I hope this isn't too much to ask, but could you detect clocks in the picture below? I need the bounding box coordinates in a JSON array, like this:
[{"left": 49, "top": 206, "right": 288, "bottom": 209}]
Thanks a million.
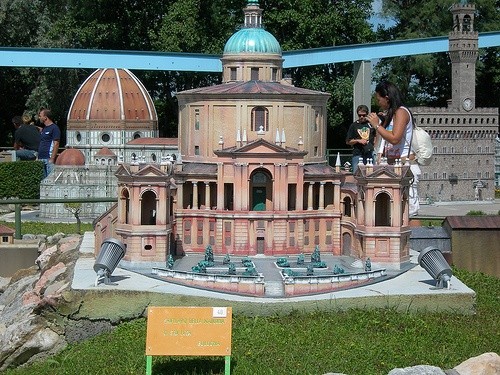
[{"left": 462, "top": 96, "right": 474, "bottom": 111}]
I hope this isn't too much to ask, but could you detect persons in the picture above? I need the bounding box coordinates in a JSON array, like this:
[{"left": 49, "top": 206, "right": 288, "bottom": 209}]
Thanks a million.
[
  {"left": 346, "top": 105, "right": 376, "bottom": 172},
  {"left": 364, "top": 80, "right": 420, "bottom": 227},
  {"left": 12, "top": 109, "right": 60, "bottom": 175}
]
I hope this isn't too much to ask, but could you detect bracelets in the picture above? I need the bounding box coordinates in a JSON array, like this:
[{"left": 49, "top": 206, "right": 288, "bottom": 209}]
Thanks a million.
[{"left": 375, "top": 124, "right": 381, "bottom": 130}]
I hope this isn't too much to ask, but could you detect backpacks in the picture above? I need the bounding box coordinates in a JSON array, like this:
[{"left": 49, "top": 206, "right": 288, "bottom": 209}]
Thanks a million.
[{"left": 393, "top": 123, "right": 433, "bottom": 166}]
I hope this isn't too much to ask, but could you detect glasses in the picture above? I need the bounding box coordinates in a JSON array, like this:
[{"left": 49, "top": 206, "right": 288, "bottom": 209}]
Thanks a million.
[{"left": 358, "top": 114, "right": 366, "bottom": 117}]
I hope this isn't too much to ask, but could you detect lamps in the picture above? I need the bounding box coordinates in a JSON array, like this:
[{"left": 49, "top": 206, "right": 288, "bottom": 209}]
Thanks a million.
[
  {"left": 418, "top": 246, "right": 454, "bottom": 291},
  {"left": 92, "top": 236, "right": 127, "bottom": 288}
]
